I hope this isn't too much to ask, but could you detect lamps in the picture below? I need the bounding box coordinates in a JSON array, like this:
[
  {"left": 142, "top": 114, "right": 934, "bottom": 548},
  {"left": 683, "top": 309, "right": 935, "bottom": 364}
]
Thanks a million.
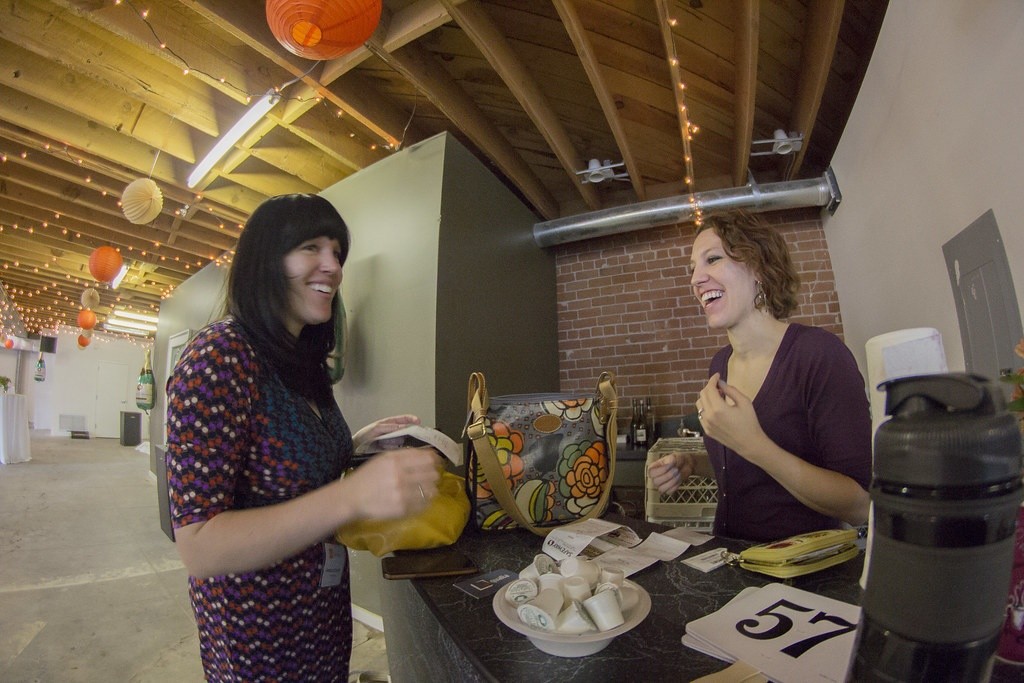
[
  {"left": 112, "top": 261, "right": 131, "bottom": 290},
  {"left": 185, "top": 86, "right": 281, "bottom": 189}
]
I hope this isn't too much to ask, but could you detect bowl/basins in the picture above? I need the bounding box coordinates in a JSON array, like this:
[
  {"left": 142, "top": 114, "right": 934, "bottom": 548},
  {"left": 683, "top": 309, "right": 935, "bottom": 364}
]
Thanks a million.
[{"left": 491, "top": 577, "right": 651, "bottom": 658}]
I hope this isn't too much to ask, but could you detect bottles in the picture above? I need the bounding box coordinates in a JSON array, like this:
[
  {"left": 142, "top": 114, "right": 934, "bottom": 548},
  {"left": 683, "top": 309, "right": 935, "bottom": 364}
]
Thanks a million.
[
  {"left": 34, "top": 351, "right": 46, "bottom": 381},
  {"left": 325, "top": 287, "right": 349, "bottom": 385},
  {"left": 645, "top": 398, "right": 656, "bottom": 446},
  {"left": 631, "top": 398, "right": 638, "bottom": 445},
  {"left": 136, "top": 349, "right": 156, "bottom": 410},
  {"left": 842, "top": 371, "right": 1023, "bottom": 683},
  {"left": 637, "top": 399, "right": 647, "bottom": 446}
]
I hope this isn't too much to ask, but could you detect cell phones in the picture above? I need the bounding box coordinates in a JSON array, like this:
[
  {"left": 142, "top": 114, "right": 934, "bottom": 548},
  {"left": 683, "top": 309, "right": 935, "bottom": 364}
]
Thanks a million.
[{"left": 381, "top": 552, "right": 477, "bottom": 580}]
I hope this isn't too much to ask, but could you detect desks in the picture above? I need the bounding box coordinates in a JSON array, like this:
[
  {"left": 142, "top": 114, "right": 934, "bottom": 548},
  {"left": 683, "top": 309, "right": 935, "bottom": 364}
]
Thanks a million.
[{"left": 0, "top": 391, "right": 32, "bottom": 465}]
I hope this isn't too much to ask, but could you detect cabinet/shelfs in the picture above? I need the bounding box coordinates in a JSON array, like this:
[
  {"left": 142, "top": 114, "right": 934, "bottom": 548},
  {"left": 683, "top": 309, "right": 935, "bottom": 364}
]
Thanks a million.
[{"left": 154, "top": 445, "right": 176, "bottom": 542}]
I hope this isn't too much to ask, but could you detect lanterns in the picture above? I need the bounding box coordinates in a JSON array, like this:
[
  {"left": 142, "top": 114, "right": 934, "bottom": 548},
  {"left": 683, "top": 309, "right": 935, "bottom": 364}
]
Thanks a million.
[
  {"left": 5, "top": 339, "right": 15, "bottom": 349},
  {"left": 78, "top": 335, "right": 91, "bottom": 347},
  {"left": 265, "top": 0, "right": 382, "bottom": 60},
  {"left": 78, "top": 310, "right": 96, "bottom": 329},
  {"left": 88, "top": 247, "right": 122, "bottom": 282}
]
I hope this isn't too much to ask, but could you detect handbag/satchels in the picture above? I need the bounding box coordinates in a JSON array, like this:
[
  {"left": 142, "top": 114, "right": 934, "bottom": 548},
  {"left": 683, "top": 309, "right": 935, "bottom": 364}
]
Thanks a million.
[
  {"left": 461, "top": 371, "right": 622, "bottom": 530},
  {"left": 335, "top": 470, "right": 469, "bottom": 556}
]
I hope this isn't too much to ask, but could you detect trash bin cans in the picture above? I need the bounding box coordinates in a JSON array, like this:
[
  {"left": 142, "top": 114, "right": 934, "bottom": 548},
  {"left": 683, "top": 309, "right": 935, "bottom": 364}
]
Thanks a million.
[{"left": 120, "top": 411, "right": 142, "bottom": 446}]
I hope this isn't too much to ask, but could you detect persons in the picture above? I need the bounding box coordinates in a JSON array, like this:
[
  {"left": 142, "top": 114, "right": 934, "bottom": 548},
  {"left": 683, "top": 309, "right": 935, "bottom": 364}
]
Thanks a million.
[
  {"left": 167, "top": 189, "right": 448, "bottom": 683},
  {"left": 648, "top": 207, "right": 873, "bottom": 542}
]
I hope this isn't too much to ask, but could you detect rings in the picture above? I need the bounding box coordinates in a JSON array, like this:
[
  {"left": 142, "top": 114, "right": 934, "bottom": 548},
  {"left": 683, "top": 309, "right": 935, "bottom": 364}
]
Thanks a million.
[{"left": 698, "top": 408, "right": 705, "bottom": 420}]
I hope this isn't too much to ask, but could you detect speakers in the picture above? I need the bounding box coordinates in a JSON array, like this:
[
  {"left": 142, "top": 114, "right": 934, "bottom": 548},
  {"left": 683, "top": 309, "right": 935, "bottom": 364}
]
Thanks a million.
[{"left": 120, "top": 411, "right": 142, "bottom": 446}]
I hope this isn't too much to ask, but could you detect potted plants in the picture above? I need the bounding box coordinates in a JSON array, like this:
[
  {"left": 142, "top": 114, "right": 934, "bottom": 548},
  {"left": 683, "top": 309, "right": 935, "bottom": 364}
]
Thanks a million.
[{"left": 0, "top": 375, "right": 12, "bottom": 394}]
